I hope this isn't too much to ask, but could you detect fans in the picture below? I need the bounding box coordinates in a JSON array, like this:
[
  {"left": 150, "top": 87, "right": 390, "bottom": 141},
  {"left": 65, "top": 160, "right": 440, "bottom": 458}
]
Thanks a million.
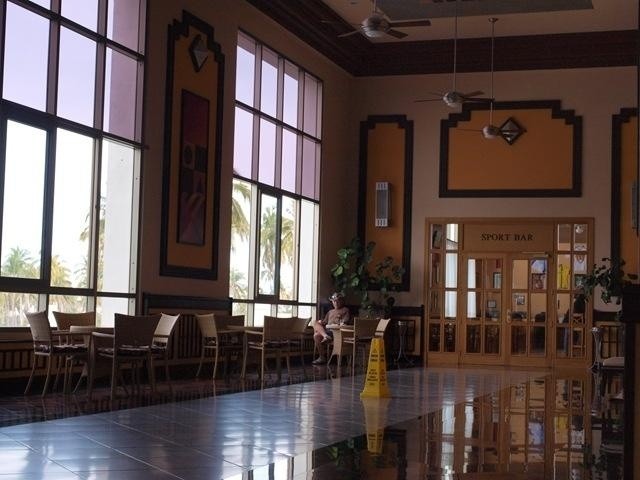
[
  {"left": 414, "top": 10, "right": 495, "bottom": 107},
  {"left": 323, "top": 0, "right": 432, "bottom": 39},
  {"left": 457, "top": 18, "right": 527, "bottom": 139}
]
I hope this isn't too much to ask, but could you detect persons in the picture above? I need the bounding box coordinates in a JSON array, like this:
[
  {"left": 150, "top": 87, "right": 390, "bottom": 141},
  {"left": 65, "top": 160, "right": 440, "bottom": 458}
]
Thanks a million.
[{"left": 310, "top": 293, "right": 350, "bottom": 365}]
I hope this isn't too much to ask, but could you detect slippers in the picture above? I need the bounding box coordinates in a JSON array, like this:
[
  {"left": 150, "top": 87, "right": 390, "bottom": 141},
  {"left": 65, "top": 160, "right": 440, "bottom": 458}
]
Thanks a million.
[{"left": 311, "top": 336, "right": 333, "bottom": 365}]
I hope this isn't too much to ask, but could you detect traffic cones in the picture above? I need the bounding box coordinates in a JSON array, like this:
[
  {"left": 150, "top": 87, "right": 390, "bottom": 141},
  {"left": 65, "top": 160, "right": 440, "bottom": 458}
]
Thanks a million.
[
  {"left": 357, "top": 400, "right": 391, "bottom": 457},
  {"left": 359, "top": 337, "right": 393, "bottom": 400}
]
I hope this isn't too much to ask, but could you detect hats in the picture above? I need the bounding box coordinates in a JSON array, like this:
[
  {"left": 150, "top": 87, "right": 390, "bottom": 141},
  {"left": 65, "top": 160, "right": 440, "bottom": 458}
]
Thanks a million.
[{"left": 328, "top": 291, "right": 345, "bottom": 302}]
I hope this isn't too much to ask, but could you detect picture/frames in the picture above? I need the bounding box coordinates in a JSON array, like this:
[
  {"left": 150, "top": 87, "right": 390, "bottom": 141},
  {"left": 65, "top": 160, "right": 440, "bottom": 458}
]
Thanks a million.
[{"left": 176, "top": 88, "right": 210, "bottom": 246}]
